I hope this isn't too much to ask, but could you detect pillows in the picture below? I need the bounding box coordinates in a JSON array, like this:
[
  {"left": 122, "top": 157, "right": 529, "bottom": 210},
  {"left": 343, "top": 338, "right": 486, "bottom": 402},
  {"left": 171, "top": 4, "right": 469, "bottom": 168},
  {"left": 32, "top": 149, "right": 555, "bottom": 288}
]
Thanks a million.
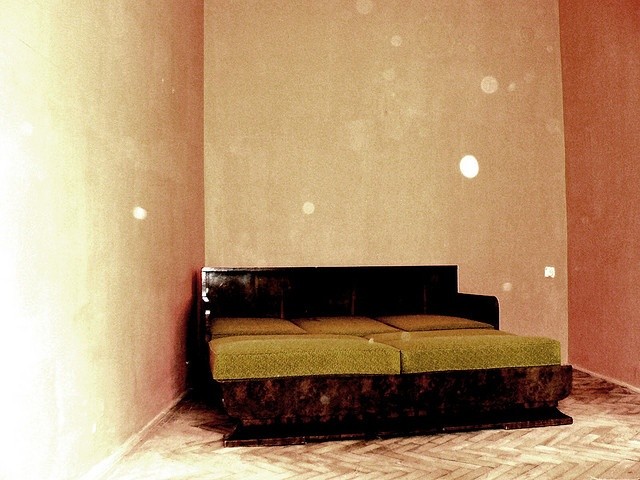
[
  {"left": 292, "top": 315, "right": 400, "bottom": 335},
  {"left": 210, "top": 315, "right": 307, "bottom": 336},
  {"left": 375, "top": 313, "right": 494, "bottom": 332}
]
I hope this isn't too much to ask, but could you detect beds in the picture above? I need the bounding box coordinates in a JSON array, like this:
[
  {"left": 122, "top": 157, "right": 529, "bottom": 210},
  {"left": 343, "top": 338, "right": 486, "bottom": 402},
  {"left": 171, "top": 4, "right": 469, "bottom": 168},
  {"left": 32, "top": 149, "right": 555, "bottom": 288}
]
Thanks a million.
[{"left": 198, "top": 265, "right": 575, "bottom": 447}]
